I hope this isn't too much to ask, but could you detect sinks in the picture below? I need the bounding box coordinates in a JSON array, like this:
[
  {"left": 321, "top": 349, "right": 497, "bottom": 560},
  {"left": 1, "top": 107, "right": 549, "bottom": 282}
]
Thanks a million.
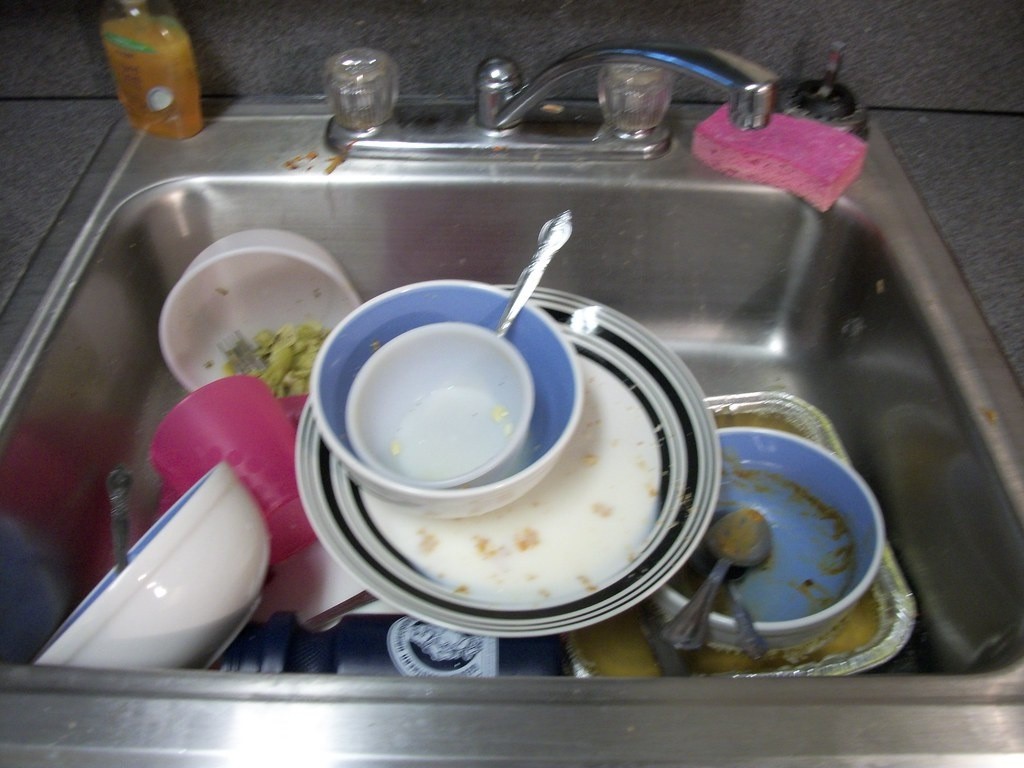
[{"left": 0, "top": 141, "right": 1024, "bottom": 719}]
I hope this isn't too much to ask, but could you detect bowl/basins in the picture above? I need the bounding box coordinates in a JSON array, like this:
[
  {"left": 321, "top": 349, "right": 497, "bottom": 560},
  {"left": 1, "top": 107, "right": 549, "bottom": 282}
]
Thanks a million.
[
  {"left": 343, "top": 321, "right": 533, "bottom": 493},
  {"left": 310, "top": 277, "right": 584, "bottom": 520},
  {"left": 157, "top": 227, "right": 360, "bottom": 399},
  {"left": 644, "top": 428, "right": 886, "bottom": 657},
  {"left": 25, "top": 459, "right": 274, "bottom": 674}
]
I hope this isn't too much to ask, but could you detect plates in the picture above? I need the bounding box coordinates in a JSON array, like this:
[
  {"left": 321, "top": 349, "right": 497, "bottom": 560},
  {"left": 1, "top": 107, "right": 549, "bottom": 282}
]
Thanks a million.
[{"left": 295, "top": 283, "right": 721, "bottom": 641}]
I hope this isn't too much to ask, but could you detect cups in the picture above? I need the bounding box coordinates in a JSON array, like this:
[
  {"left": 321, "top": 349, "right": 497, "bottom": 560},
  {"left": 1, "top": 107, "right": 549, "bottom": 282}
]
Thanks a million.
[{"left": 148, "top": 375, "right": 322, "bottom": 554}]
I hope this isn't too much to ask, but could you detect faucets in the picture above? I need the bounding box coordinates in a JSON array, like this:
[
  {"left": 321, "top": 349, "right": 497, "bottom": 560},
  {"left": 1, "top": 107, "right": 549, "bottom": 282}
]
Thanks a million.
[{"left": 472, "top": 38, "right": 782, "bottom": 143}]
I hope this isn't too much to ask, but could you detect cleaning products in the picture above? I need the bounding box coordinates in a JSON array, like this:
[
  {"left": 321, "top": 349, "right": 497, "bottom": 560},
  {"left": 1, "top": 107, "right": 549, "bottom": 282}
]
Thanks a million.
[{"left": 99, "top": 0, "right": 206, "bottom": 140}]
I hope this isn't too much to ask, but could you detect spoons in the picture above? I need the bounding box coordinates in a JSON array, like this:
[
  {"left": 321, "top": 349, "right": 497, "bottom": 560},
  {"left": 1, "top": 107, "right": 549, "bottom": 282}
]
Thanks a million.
[{"left": 655, "top": 507, "right": 770, "bottom": 653}]
